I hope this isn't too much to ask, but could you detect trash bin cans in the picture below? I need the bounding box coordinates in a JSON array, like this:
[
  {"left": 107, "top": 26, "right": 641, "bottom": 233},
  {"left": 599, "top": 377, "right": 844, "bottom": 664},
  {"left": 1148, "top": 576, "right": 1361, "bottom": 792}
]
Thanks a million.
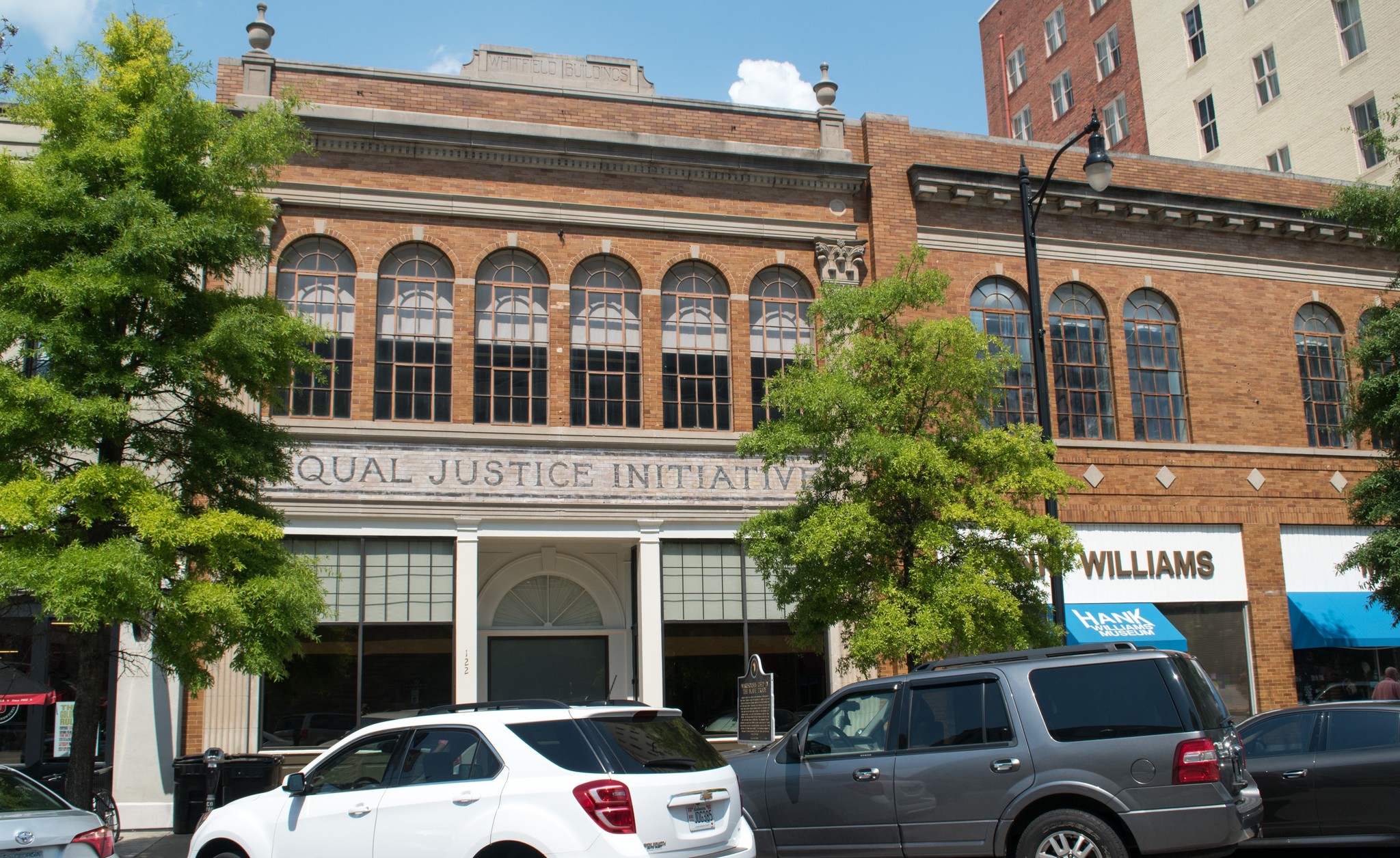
[
  {"left": 172, "top": 753, "right": 224, "bottom": 834},
  {"left": 220, "top": 752, "right": 285, "bottom": 806}
]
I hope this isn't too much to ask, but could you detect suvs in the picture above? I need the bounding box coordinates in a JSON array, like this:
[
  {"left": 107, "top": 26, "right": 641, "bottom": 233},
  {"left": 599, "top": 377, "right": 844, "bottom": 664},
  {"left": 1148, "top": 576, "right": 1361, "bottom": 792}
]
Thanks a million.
[
  {"left": 720, "top": 639, "right": 1265, "bottom": 858},
  {"left": 183, "top": 699, "right": 761, "bottom": 858}
]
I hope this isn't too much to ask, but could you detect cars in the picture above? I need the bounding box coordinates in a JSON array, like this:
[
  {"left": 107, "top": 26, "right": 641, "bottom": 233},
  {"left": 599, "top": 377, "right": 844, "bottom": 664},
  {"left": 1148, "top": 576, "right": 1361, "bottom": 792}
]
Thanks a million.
[
  {"left": 1305, "top": 680, "right": 1382, "bottom": 702},
  {"left": 1, "top": 763, "right": 121, "bottom": 858},
  {"left": 1217, "top": 699, "right": 1400, "bottom": 858}
]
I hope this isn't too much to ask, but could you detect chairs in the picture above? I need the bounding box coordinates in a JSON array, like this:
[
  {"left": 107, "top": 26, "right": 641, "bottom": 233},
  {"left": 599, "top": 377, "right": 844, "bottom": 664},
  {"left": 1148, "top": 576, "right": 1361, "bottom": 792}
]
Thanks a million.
[{"left": 911, "top": 698, "right": 935, "bottom": 748}]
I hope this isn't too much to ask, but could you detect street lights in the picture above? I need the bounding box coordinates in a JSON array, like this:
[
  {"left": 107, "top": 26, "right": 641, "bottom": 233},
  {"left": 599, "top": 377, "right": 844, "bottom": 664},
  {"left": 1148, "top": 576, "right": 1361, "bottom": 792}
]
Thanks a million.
[{"left": 1017, "top": 107, "right": 1117, "bottom": 645}]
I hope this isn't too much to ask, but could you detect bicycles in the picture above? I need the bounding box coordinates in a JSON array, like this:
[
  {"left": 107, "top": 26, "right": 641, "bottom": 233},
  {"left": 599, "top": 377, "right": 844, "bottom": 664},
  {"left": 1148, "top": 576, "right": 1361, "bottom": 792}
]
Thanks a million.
[{"left": 42, "top": 769, "right": 121, "bottom": 844}]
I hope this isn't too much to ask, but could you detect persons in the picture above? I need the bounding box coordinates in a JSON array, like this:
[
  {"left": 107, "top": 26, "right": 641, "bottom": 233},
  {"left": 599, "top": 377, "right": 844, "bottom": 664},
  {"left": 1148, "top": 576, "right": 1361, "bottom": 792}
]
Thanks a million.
[{"left": 1371, "top": 667, "right": 1400, "bottom": 700}]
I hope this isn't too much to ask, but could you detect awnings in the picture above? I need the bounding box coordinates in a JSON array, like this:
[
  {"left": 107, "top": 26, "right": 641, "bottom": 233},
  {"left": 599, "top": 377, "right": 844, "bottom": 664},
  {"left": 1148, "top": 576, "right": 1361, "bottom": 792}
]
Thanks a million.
[
  {"left": 1287, "top": 592, "right": 1400, "bottom": 649},
  {"left": 1047, "top": 603, "right": 1188, "bottom": 652}
]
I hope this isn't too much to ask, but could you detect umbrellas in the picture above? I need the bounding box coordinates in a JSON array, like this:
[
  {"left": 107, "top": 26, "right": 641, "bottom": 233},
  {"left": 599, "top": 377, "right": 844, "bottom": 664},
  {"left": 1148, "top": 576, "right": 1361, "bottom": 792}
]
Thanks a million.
[{"left": 0, "top": 661, "right": 56, "bottom": 705}]
